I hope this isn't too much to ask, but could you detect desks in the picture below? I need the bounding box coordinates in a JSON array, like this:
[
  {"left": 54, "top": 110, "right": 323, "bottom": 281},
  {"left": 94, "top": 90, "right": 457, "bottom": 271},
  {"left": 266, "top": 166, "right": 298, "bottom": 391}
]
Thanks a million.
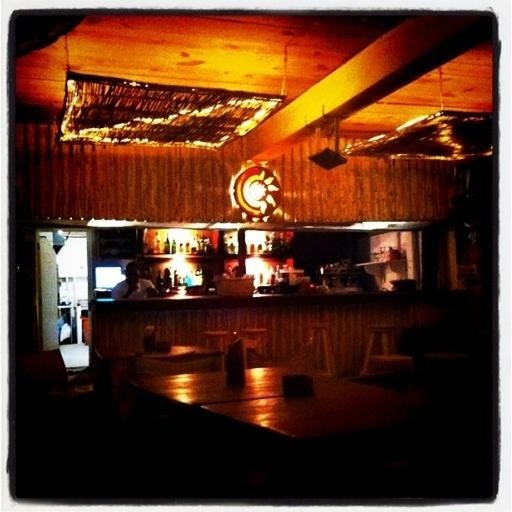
[
  {"left": 127, "top": 366, "right": 301, "bottom": 407},
  {"left": 201, "top": 382, "right": 416, "bottom": 440},
  {"left": 139, "top": 345, "right": 196, "bottom": 360}
]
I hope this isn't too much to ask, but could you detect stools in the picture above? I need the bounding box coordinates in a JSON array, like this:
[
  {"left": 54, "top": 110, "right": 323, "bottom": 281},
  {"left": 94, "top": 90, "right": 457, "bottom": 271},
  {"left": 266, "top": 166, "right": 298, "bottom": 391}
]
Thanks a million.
[
  {"left": 203, "top": 322, "right": 337, "bottom": 381},
  {"left": 363, "top": 324, "right": 400, "bottom": 372}
]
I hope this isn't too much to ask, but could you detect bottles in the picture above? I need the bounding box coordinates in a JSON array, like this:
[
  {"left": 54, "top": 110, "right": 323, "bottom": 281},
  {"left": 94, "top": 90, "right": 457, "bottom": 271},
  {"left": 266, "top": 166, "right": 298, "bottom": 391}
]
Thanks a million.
[
  {"left": 156, "top": 267, "right": 179, "bottom": 291},
  {"left": 153, "top": 231, "right": 190, "bottom": 255},
  {"left": 276, "top": 264, "right": 296, "bottom": 285}
]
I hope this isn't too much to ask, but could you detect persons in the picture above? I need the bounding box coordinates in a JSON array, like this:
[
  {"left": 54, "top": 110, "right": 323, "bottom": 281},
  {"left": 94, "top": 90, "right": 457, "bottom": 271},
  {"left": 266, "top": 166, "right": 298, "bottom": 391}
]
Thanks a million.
[
  {"left": 111, "top": 261, "right": 160, "bottom": 300},
  {"left": 232, "top": 266, "right": 243, "bottom": 278}
]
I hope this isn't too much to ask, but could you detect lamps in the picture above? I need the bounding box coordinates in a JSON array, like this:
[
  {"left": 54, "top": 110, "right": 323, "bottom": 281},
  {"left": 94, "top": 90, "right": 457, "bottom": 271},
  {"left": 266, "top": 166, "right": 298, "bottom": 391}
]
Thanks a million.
[{"left": 232, "top": 167, "right": 282, "bottom": 219}]
